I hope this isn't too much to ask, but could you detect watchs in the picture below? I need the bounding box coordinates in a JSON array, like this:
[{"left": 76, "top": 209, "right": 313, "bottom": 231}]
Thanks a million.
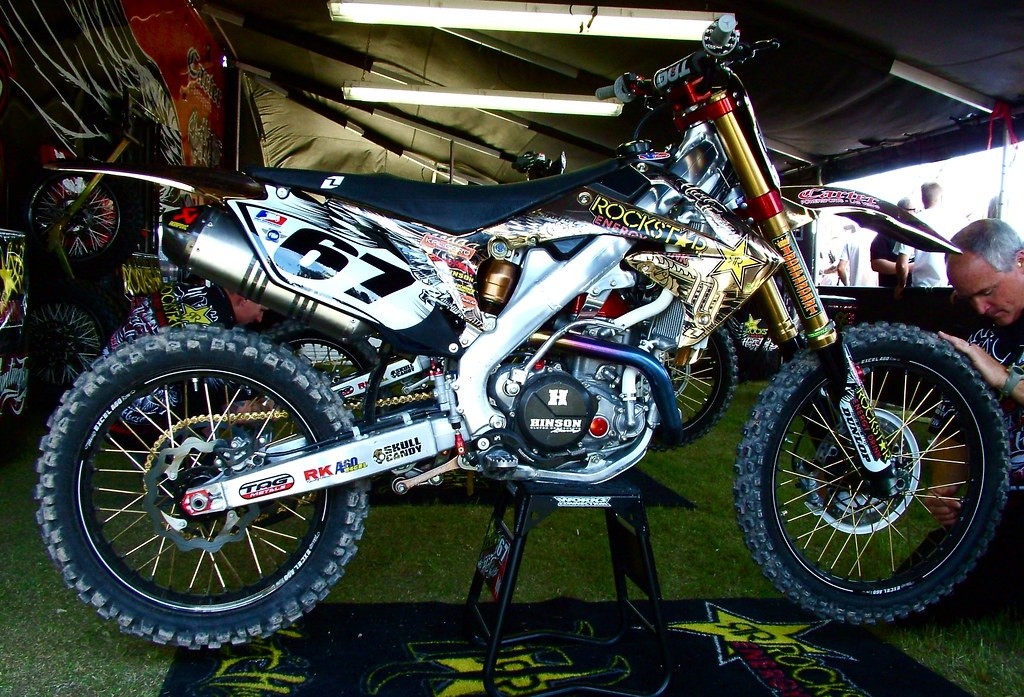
[{"left": 999, "top": 364, "right": 1024, "bottom": 398}]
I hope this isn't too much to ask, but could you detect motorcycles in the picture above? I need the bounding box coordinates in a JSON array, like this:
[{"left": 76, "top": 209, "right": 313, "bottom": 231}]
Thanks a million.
[{"left": 29, "top": 8, "right": 1013, "bottom": 697}]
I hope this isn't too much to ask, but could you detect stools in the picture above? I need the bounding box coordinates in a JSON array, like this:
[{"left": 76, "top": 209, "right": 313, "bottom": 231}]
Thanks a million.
[{"left": 460, "top": 465, "right": 698, "bottom": 697}]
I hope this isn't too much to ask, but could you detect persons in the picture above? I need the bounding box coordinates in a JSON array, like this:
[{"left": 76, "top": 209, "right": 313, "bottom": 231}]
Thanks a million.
[
  {"left": 889, "top": 219, "right": 1024, "bottom": 625},
  {"left": 815, "top": 183, "right": 962, "bottom": 305}
]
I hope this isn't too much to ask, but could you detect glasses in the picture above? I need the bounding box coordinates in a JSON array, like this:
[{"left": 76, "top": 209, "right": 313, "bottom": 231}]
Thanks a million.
[{"left": 906, "top": 208, "right": 922, "bottom": 213}]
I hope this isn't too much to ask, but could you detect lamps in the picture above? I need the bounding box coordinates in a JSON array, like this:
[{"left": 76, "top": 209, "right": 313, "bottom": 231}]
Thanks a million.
[
  {"left": 343, "top": 23, "right": 624, "bottom": 117},
  {"left": 328, "top": 0, "right": 737, "bottom": 41}
]
